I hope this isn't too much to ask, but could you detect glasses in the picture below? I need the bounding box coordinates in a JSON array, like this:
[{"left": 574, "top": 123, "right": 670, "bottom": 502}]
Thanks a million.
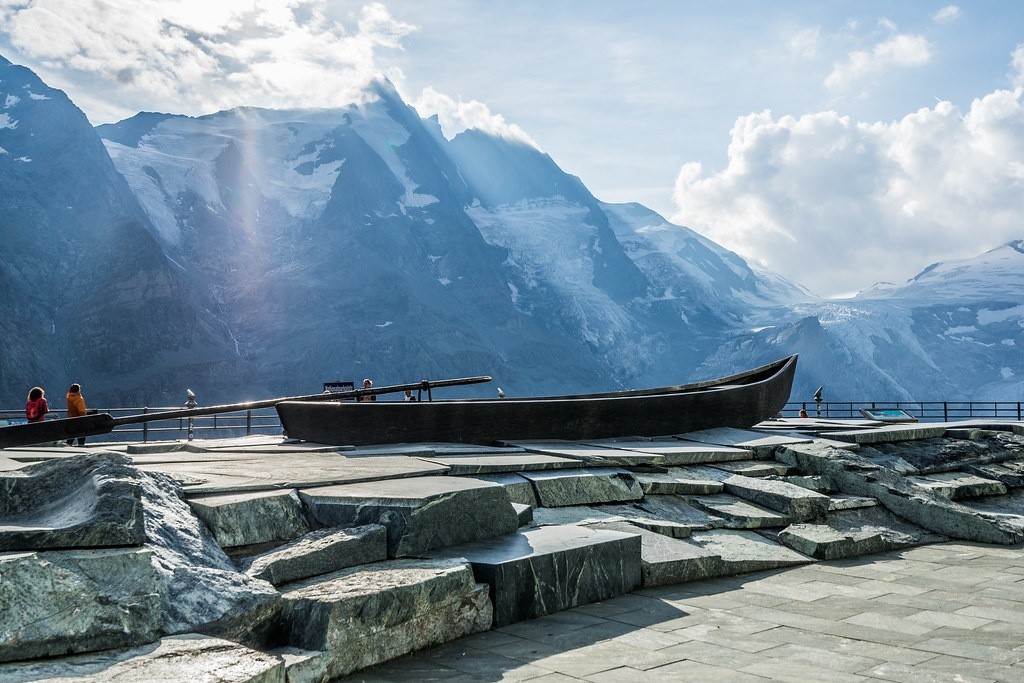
[{"left": 364, "top": 382, "right": 370, "bottom": 384}]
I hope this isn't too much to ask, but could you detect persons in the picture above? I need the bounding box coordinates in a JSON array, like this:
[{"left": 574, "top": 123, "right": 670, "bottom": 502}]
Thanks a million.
[
  {"left": 26, "top": 387, "right": 49, "bottom": 423},
  {"left": 66, "top": 384, "right": 87, "bottom": 446},
  {"left": 404, "top": 390, "right": 416, "bottom": 401},
  {"left": 799, "top": 410, "right": 808, "bottom": 418},
  {"left": 360, "top": 379, "right": 376, "bottom": 401}
]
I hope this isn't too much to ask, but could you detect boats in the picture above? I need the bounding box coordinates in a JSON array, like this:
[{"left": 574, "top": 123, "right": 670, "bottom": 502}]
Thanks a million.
[{"left": 273, "top": 351, "right": 802, "bottom": 446}]
